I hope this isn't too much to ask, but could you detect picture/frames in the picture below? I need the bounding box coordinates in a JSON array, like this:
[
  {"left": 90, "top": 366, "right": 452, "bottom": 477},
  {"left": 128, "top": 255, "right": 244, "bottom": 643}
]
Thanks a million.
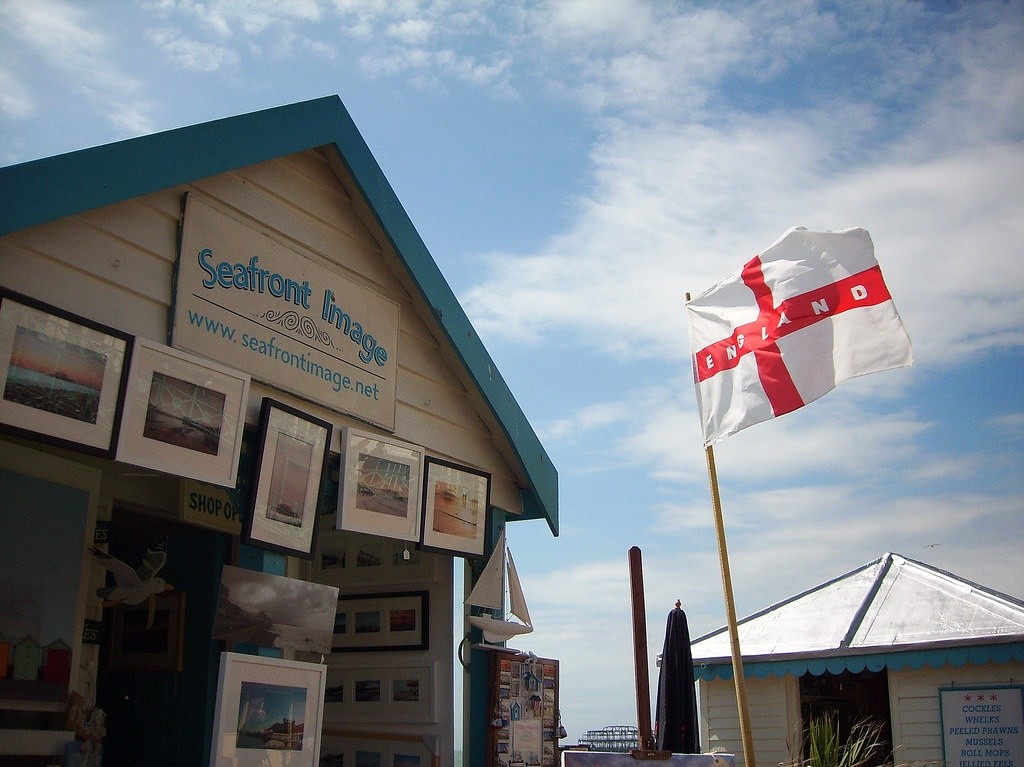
[
  {"left": 0, "top": 286, "right": 137, "bottom": 461},
  {"left": 336, "top": 426, "right": 425, "bottom": 543},
  {"left": 304, "top": 530, "right": 440, "bottom": 588},
  {"left": 240, "top": 397, "right": 334, "bottom": 562},
  {"left": 322, "top": 660, "right": 438, "bottom": 724},
  {"left": 105, "top": 589, "right": 187, "bottom": 675},
  {"left": 330, "top": 590, "right": 430, "bottom": 652},
  {"left": 209, "top": 651, "right": 328, "bottom": 767},
  {"left": 485, "top": 651, "right": 559, "bottom": 767},
  {"left": 318, "top": 728, "right": 441, "bottom": 767},
  {"left": 416, "top": 455, "right": 491, "bottom": 563},
  {"left": 112, "top": 335, "right": 250, "bottom": 489}
]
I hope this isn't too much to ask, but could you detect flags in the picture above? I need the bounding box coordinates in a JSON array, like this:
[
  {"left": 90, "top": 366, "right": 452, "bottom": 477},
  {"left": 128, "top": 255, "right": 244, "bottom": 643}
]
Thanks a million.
[{"left": 686, "top": 222, "right": 912, "bottom": 450}]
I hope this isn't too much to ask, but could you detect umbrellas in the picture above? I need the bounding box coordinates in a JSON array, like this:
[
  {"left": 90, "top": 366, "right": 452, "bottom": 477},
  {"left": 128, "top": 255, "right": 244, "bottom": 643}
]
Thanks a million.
[{"left": 655, "top": 600, "right": 700, "bottom": 755}]
